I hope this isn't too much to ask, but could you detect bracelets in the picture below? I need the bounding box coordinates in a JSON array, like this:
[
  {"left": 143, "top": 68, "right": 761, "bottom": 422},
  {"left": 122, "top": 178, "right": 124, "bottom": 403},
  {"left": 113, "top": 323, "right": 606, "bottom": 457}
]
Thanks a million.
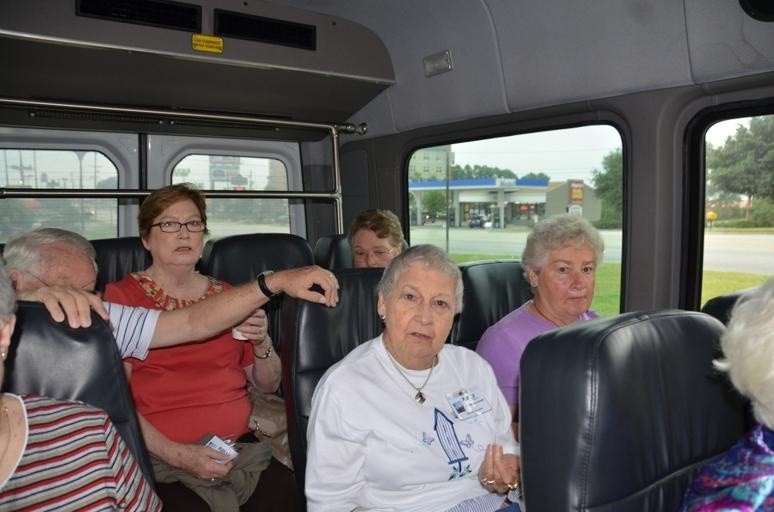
[{"left": 253, "top": 345, "right": 274, "bottom": 359}]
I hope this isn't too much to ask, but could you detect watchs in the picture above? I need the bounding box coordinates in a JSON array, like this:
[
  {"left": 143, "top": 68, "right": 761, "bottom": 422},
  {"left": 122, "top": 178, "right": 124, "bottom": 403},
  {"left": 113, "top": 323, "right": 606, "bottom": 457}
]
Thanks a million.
[{"left": 256, "top": 270, "right": 281, "bottom": 301}]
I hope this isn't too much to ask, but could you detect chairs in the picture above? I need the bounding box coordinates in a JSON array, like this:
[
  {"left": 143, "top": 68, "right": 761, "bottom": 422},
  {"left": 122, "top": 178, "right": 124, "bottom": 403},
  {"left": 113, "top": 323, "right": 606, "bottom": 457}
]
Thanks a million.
[
  {"left": 4, "top": 300, "right": 158, "bottom": 492},
  {"left": 703, "top": 289, "right": 747, "bottom": 323},
  {"left": 90, "top": 237, "right": 151, "bottom": 292},
  {"left": 454, "top": 259, "right": 534, "bottom": 351},
  {"left": 520, "top": 307, "right": 755, "bottom": 512},
  {"left": 202, "top": 234, "right": 315, "bottom": 352},
  {"left": 314, "top": 236, "right": 410, "bottom": 267},
  {"left": 280, "top": 268, "right": 454, "bottom": 481}
]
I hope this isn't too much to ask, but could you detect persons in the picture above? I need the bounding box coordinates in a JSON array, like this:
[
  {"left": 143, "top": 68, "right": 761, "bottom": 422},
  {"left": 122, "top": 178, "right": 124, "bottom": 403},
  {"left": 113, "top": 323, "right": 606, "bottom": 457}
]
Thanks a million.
[
  {"left": 680, "top": 275, "right": 774, "bottom": 512},
  {"left": 303, "top": 243, "right": 527, "bottom": 512},
  {"left": 473, "top": 211, "right": 606, "bottom": 442},
  {"left": 346, "top": 205, "right": 404, "bottom": 269},
  {"left": 0, "top": 255, "right": 164, "bottom": 512},
  {"left": 100, "top": 182, "right": 304, "bottom": 512},
  {"left": 2, "top": 227, "right": 340, "bottom": 363}
]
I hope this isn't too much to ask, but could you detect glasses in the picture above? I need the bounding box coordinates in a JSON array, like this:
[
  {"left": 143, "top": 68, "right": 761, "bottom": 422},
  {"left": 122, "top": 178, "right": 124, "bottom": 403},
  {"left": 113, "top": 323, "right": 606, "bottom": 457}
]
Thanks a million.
[{"left": 151, "top": 219, "right": 206, "bottom": 233}]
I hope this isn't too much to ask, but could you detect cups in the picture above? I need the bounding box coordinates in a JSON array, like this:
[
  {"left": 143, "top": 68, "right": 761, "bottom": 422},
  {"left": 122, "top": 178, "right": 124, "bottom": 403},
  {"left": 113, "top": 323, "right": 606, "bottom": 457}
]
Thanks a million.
[{"left": 231, "top": 320, "right": 250, "bottom": 342}]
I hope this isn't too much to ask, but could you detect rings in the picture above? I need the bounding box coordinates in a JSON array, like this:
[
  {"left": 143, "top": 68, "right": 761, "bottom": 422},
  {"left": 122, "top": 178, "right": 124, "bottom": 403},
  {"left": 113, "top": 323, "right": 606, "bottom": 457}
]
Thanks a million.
[
  {"left": 211, "top": 478, "right": 214, "bottom": 482},
  {"left": 507, "top": 483, "right": 519, "bottom": 491},
  {"left": 487, "top": 480, "right": 496, "bottom": 488},
  {"left": 480, "top": 478, "right": 488, "bottom": 487},
  {"left": 328, "top": 273, "right": 334, "bottom": 278}
]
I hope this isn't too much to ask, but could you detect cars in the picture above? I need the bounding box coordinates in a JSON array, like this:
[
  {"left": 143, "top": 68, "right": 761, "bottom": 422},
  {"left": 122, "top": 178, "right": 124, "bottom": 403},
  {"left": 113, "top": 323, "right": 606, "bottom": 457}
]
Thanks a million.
[{"left": 469, "top": 216, "right": 485, "bottom": 228}]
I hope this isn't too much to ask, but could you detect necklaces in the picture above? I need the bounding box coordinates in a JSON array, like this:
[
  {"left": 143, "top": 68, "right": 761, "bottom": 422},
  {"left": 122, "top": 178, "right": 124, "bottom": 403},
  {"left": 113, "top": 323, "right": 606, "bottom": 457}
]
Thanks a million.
[{"left": 381, "top": 333, "right": 437, "bottom": 404}]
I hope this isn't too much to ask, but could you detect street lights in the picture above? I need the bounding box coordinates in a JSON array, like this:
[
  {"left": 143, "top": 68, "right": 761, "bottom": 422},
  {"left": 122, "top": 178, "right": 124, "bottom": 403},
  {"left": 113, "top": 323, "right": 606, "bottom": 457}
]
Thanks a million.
[{"left": 75, "top": 151, "right": 88, "bottom": 236}]
